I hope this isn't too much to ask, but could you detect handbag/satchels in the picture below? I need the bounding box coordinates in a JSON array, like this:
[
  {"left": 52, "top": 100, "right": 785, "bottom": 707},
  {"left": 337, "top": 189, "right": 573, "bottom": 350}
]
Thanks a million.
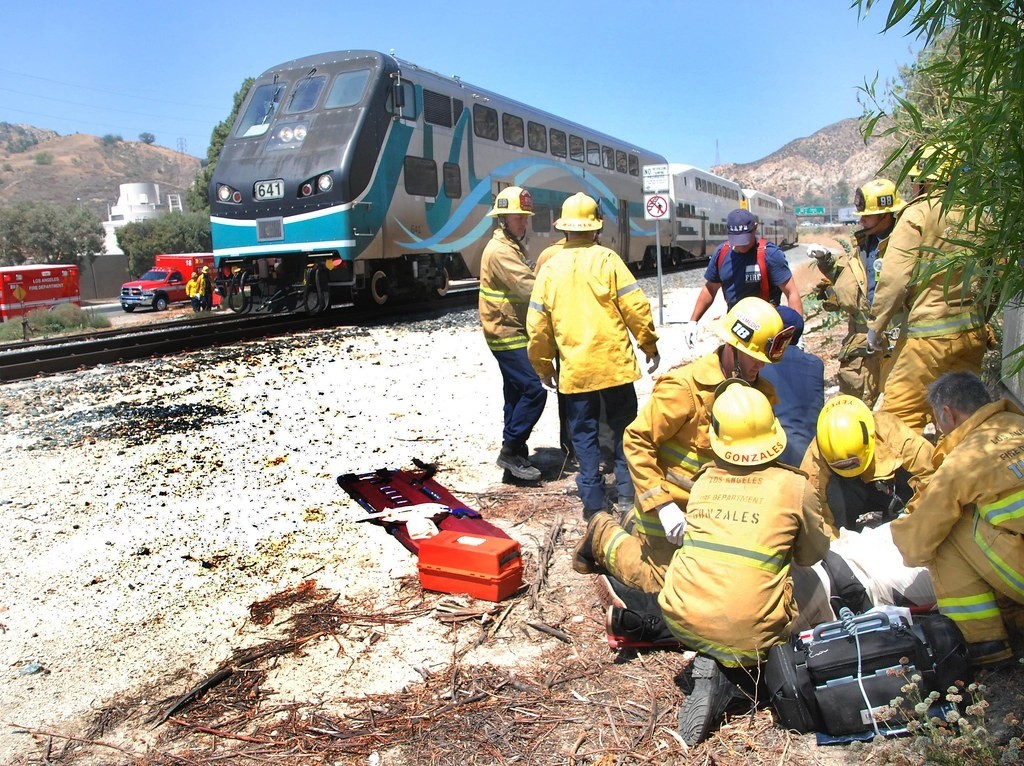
[{"left": 762, "top": 612, "right": 977, "bottom": 745}]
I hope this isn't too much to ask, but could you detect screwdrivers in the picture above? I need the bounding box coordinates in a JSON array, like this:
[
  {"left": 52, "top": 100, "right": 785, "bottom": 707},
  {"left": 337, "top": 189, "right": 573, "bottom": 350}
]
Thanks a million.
[
  {"left": 350, "top": 491, "right": 374, "bottom": 513},
  {"left": 395, "top": 468, "right": 441, "bottom": 500}
]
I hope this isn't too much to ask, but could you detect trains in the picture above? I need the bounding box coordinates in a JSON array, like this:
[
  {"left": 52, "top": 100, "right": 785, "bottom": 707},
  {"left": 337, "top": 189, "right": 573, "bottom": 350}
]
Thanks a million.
[
  {"left": 837, "top": 207, "right": 859, "bottom": 225},
  {"left": 209, "top": 49, "right": 799, "bottom": 320}
]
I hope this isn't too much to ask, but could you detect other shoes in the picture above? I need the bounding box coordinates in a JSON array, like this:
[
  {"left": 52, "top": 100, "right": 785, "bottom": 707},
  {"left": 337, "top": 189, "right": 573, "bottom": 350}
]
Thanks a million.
[
  {"left": 968, "top": 641, "right": 1014, "bottom": 666},
  {"left": 497, "top": 434, "right": 685, "bottom": 647}
]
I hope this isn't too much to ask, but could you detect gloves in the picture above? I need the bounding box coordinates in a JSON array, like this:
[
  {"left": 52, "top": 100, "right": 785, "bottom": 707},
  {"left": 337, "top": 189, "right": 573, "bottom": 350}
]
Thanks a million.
[
  {"left": 684, "top": 321, "right": 697, "bottom": 350},
  {"left": 655, "top": 500, "right": 687, "bottom": 547},
  {"left": 806, "top": 243, "right": 828, "bottom": 258},
  {"left": 866, "top": 329, "right": 885, "bottom": 350},
  {"left": 540, "top": 367, "right": 560, "bottom": 394},
  {"left": 646, "top": 352, "right": 660, "bottom": 374}
]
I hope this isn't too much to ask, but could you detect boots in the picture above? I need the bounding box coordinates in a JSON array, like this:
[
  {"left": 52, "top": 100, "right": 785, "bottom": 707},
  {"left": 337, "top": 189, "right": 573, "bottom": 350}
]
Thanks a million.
[{"left": 679, "top": 651, "right": 769, "bottom": 747}]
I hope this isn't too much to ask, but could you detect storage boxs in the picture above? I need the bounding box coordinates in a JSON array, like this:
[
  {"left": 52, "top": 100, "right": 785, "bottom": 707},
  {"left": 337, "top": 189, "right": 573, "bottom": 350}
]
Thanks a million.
[{"left": 418, "top": 528, "right": 525, "bottom": 603}]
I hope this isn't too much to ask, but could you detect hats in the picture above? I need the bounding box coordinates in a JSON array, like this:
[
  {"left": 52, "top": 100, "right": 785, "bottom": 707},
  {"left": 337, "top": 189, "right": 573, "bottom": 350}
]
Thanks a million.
[
  {"left": 725, "top": 209, "right": 755, "bottom": 247},
  {"left": 775, "top": 305, "right": 805, "bottom": 345}
]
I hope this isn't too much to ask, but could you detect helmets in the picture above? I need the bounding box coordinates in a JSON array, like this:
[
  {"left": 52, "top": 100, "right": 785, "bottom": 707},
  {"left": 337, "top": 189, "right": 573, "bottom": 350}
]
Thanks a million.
[
  {"left": 708, "top": 377, "right": 787, "bottom": 467},
  {"left": 707, "top": 297, "right": 795, "bottom": 364},
  {"left": 554, "top": 192, "right": 603, "bottom": 232},
  {"left": 485, "top": 185, "right": 536, "bottom": 217},
  {"left": 201, "top": 266, "right": 210, "bottom": 274},
  {"left": 852, "top": 179, "right": 909, "bottom": 216},
  {"left": 904, "top": 140, "right": 963, "bottom": 182},
  {"left": 191, "top": 272, "right": 198, "bottom": 279},
  {"left": 816, "top": 395, "right": 875, "bottom": 478}
]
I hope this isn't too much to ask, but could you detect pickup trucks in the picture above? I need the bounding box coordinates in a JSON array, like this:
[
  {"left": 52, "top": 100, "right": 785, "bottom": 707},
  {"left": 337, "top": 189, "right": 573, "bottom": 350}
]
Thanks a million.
[{"left": 120, "top": 253, "right": 230, "bottom": 313}]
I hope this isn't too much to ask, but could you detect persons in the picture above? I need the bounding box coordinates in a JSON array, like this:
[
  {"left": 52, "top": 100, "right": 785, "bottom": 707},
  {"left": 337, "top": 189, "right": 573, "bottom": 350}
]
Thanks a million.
[
  {"left": 686, "top": 209, "right": 804, "bottom": 350},
  {"left": 889, "top": 372, "right": 1023, "bottom": 668},
  {"left": 806, "top": 178, "right": 907, "bottom": 398},
  {"left": 527, "top": 193, "right": 662, "bottom": 523},
  {"left": 867, "top": 143, "right": 1005, "bottom": 438},
  {"left": 572, "top": 297, "right": 829, "bottom": 738},
  {"left": 478, "top": 188, "right": 542, "bottom": 480},
  {"left": 186, "top": 266, "right": 214, "bottom": 313},
  {"left": 800, "top": 394, "right": 935, "bottom": 539},
  {"left": 760, "top": 305, "right": 823, "bottom": 468}
]
